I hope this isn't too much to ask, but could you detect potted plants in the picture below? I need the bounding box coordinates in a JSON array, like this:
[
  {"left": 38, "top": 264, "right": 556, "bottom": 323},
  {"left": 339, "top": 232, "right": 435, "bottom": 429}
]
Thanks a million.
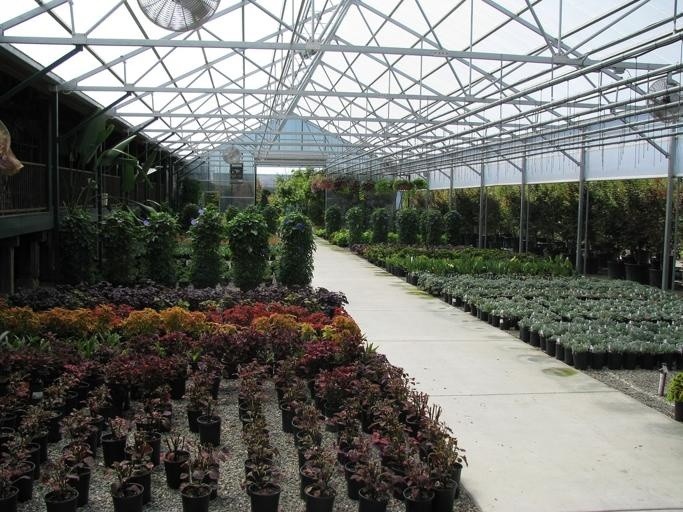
[
  {"left": 172, "top": 242, "right": 277, "bottom": 262},
  {"left": 473, "top": 180, "right": 683, "bottom": 291},
  {"left": 666, "top": 373, "right": 683, "bottom": 423}
]
